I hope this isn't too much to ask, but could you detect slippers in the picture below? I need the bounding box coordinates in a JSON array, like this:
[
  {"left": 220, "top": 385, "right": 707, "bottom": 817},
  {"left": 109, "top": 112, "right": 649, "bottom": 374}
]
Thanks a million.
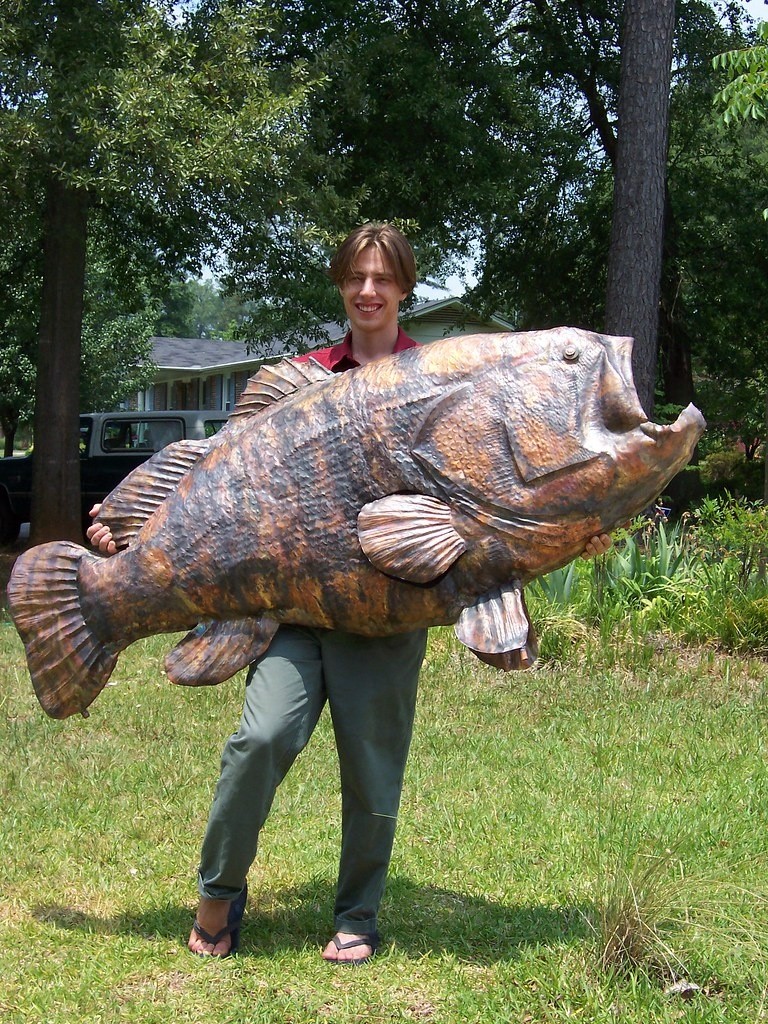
[
  {"left": 193, "top": 876, "right": 248, "bottom": 959},
  {"left": 323, "top": 934, "right": 378, "bottom": 967}
]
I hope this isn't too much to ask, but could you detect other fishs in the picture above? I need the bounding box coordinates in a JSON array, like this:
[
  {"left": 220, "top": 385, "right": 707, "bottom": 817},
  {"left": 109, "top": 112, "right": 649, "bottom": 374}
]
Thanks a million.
[{"left": 6, "top": 326, "right": 707, "bottom": 719}]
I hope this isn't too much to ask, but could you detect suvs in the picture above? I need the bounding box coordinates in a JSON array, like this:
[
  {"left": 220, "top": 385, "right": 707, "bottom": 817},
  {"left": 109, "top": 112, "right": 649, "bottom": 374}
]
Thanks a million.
[{"left": 0, "top": 407, "right": 234, "bottom": 550}]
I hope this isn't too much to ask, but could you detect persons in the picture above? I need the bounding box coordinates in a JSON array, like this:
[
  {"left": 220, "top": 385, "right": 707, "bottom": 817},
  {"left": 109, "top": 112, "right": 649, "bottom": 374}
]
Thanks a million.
[{"left": 86, "top": 225, "right": 613, "bottom": 961}]
